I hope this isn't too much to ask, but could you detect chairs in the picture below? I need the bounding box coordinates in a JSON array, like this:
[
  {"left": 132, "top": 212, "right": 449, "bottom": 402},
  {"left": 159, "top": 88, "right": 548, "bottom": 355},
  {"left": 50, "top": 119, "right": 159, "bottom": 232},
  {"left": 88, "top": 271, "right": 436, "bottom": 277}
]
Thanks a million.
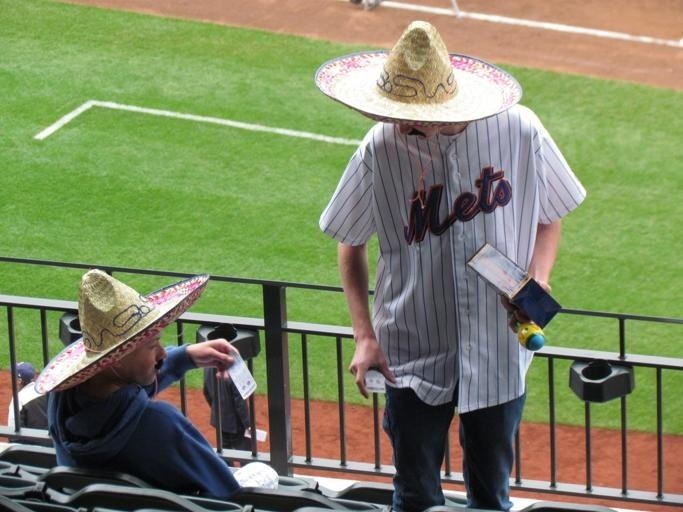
[
  {"left": 224, "top": 477, "right": 467, "bottom": 512},
  {"left": 526, "top": 501, "right": 612, "bottom": 512},
  {"left": 2, "top": 445, "right": 57, "bottom": 468},
  {"left": 0, "top": 461, "right": 241, "bottom": 512}
]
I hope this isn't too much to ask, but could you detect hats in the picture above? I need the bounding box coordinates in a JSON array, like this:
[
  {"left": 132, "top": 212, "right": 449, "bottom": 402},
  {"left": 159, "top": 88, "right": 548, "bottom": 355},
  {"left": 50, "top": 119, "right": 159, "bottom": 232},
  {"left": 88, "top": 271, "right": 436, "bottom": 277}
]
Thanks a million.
[
  {"left": 8, "top": 360, "right": 36, "bottom": 381},
  {"left": 312, "top": 19, "right": 524, "bottom": 127},
  {"left": 32, "top": 267, "right": 211, "bottom": 396}
]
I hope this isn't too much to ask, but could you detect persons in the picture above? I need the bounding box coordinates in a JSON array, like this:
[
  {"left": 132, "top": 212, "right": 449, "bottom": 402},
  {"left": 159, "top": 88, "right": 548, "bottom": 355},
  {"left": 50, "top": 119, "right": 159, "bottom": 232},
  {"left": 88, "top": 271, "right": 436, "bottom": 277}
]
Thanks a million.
[
  {"left": 31, "top": 265, "right": 254, "bottom": 510},
  {"left": 200, "top": 365, "right": 254, "bottom": 468},
  {"left": 7, "top": 361, "right": 53, "bottom": 447},
  {"left": 317, "top": 18, "right": 586, "bottom": 511}
]
